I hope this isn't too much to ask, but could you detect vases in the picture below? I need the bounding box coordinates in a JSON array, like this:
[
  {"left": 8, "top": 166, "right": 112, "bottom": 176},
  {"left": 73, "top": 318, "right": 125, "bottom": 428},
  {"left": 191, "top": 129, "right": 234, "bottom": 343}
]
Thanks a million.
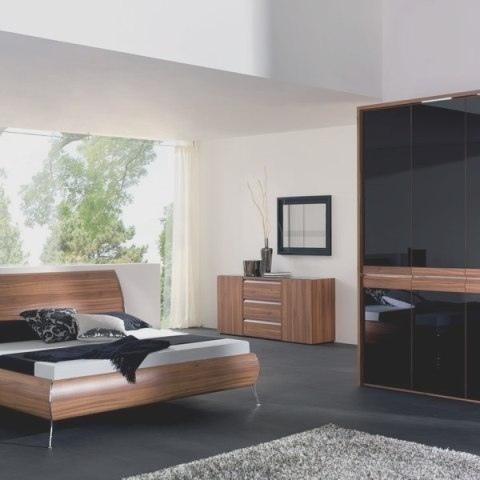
[{"left": 261, "top": 238, "right": 273, "bottom": 273}]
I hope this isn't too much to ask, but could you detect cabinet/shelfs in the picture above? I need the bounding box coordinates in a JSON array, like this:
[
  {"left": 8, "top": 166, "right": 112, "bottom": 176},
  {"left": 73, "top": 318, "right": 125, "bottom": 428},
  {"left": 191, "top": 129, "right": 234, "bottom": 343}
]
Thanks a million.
[
  {"left": 217, "top": 275, "right": 335, "bottom": 345},
  {"left": 355, "top": 89, "right": 480, "bottom": 403}
]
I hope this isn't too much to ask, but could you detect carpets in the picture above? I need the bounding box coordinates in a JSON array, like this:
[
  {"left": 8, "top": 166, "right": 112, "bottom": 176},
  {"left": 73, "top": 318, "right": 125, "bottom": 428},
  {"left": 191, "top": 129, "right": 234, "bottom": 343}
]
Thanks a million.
[{"left": 120, "top": 422, "right": 480, "bottom": 480}]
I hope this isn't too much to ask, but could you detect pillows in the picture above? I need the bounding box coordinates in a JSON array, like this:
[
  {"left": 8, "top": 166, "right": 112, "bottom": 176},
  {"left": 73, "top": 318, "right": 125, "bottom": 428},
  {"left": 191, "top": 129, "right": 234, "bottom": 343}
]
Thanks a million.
[{"left": 0, "top": 306, "right": 153, "bottom": 344}]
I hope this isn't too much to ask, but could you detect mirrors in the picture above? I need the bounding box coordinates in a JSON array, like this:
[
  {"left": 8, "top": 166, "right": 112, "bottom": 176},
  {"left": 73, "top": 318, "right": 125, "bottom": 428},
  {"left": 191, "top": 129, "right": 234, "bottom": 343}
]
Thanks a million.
[{"left": 276, "top": 195, "right": 332, "bottom": 256}]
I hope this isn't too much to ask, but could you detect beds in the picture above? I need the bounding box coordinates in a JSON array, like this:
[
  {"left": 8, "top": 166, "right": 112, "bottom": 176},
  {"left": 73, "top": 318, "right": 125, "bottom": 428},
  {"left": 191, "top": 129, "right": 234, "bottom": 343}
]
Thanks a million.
[{"left": 0, "top": 269, "right": 260, "bottom": 448}]
